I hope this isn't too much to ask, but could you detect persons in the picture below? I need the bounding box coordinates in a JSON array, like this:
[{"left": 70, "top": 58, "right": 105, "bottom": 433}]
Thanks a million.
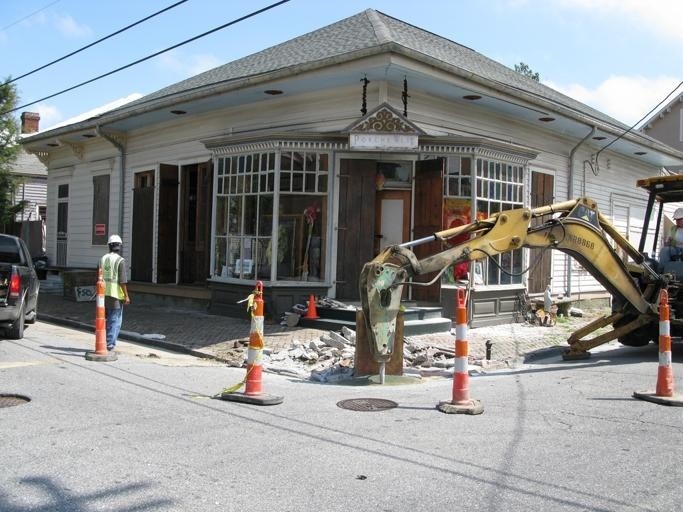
[{"left": 95, "top": 234, "right": 130, "bottom": 354}]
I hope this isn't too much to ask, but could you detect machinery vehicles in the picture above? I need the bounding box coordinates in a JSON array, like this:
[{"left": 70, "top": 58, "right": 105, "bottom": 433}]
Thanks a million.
[{"left": 355, "top": 174, "right": 683, "bottom": 377}]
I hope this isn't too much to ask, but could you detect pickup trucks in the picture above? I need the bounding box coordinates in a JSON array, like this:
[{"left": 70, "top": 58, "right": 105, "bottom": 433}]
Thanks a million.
[{"left": 0, "top": 233, "right": 46, "bottom": 341}]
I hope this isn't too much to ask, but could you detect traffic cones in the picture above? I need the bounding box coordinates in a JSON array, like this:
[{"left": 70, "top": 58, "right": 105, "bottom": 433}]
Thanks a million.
[{"left": 303, "top": 294, "right": 320, "bottom": 319}]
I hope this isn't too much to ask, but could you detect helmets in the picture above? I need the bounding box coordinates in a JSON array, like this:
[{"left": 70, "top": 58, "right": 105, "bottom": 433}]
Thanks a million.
[{"left": 107, "top": 234, "right": 123, "bottom": 245}]
